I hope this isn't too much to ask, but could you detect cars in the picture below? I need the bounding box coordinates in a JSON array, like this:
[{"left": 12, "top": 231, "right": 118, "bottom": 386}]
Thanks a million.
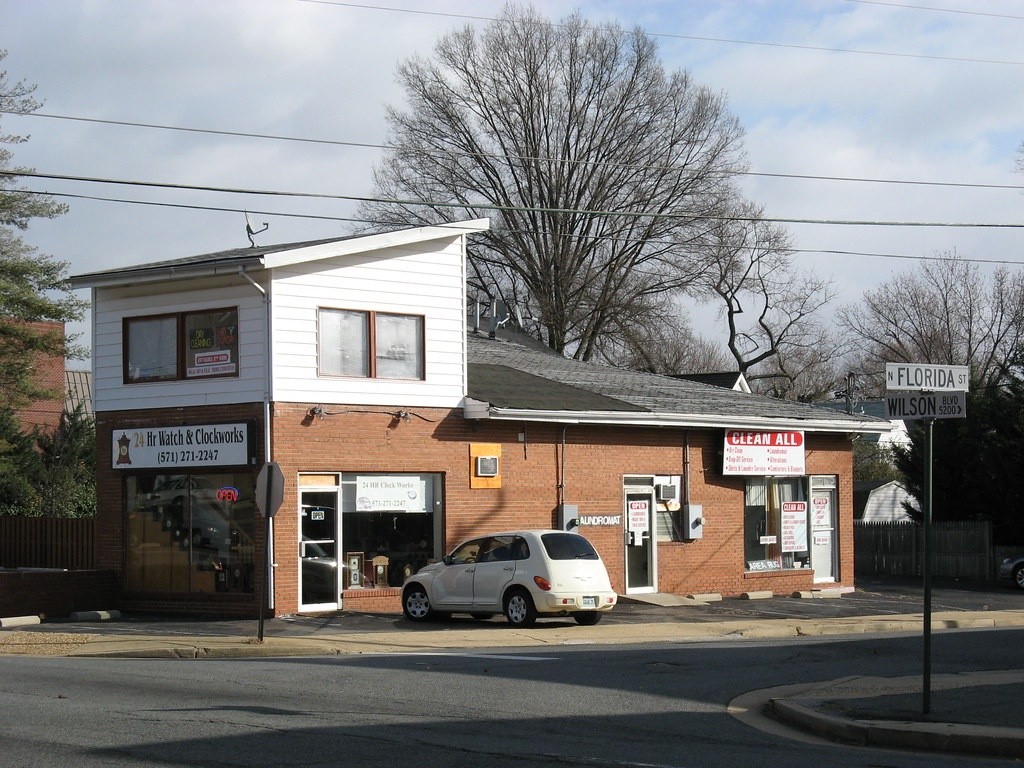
[
  {"left": 149, "top": 475, "right": 218, "bottom": 504},
  {"left": 401, "top": 529, "right": 617, "bottom": 629},
  {"left": 999, "top": 554, "right": 1024, "bottom": 590},
  {"left": 164, "top": 495, "right": 239, "bottom": 549},
  {"left": 302, "top": 535, "right": 372, "bottom": 603}
]
